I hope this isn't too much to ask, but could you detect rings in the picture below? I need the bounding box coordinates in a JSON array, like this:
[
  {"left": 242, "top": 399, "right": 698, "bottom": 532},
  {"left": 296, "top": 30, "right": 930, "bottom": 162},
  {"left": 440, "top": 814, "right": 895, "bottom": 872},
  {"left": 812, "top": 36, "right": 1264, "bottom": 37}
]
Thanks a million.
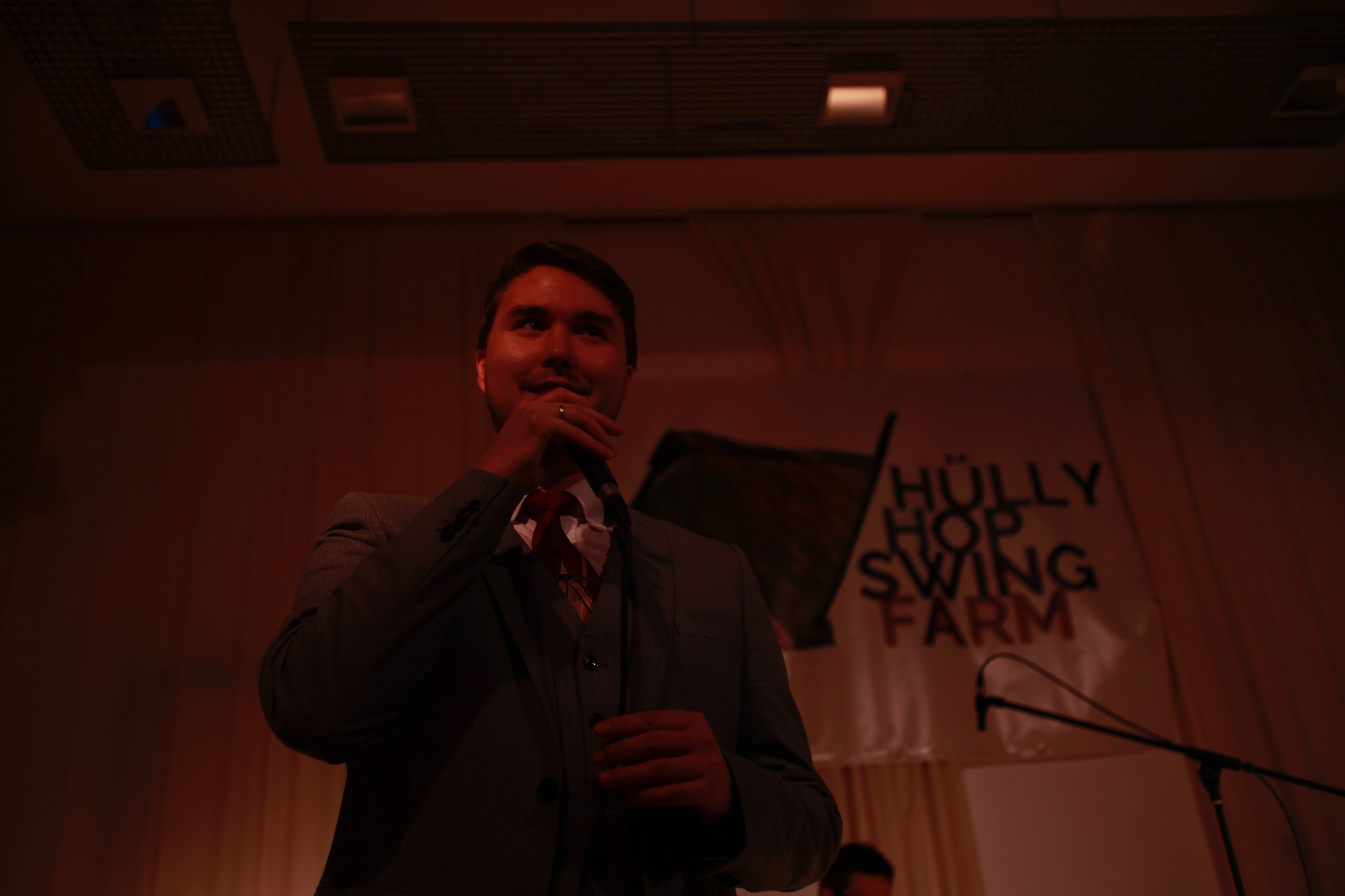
[{"left": 559, "top": 405, "right": 564, "bottom": 418}]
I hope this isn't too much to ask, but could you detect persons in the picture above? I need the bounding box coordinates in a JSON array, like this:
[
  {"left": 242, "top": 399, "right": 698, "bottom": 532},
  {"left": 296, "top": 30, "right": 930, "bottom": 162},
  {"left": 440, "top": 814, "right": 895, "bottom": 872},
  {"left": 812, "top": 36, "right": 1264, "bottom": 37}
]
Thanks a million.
[
  {"left": 257, "top": 241, "right": 842, "bottom": 896},
  {"left": 818, "top": 843, "right": 894, "bottom": 896}
]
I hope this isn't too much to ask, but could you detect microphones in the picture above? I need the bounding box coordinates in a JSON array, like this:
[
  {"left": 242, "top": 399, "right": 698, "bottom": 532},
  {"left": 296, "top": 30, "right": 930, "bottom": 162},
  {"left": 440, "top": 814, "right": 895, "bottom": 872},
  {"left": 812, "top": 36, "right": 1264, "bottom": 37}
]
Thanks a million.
[
  {"left": 975, "top": 672, "right": 986, "bottom": 730},
  {"left": 570, "top": 424, "right": 629, "bottom": 524}
]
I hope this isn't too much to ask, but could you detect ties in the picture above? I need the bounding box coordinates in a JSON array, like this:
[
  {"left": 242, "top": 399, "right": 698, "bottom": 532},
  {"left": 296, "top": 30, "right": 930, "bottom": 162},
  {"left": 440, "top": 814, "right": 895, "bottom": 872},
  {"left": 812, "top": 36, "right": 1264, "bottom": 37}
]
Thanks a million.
[{"left": 523, "top": 490, "right": 603, "bottom": 621}]
